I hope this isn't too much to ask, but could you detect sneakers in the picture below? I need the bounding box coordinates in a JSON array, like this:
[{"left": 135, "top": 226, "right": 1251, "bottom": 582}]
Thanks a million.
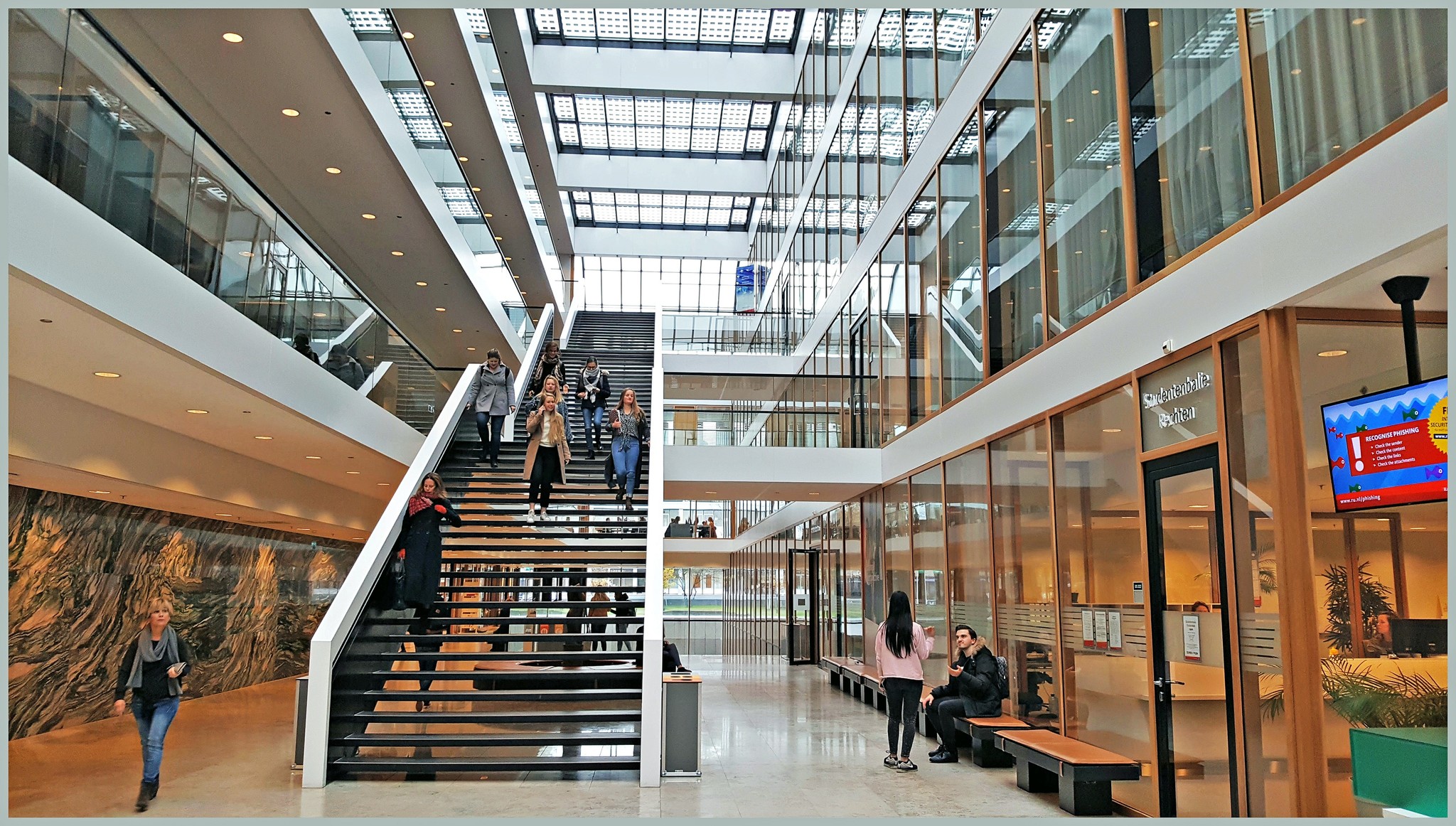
[
  {"left": 896, "top": 758, "right": 918, "bottom": 773},
  {"left": 527, "top": 509, "right": 535, "bottom": 523},
  {"left": 884, "top": 754, "right": 898, "bottom": 769},
  {"left": 540, "top": 511, "right": 551, "bottom": 521}
]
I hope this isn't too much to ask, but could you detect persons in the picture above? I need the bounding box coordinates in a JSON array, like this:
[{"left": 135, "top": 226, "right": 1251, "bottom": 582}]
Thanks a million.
[
  {"left": 1367, "top": 611, "right": 1410, "bottom": 658},
  {"left": 408, "top": 593, "right": 450, "bottom": 712},
  {"left": 574, "top": 356, "right": 611, "bottom": 460},
  {"left": 466, "top": 349, "right": 516, "bottom": 467},
  {"left": 636, "top": 624, "right": 692, "bottom": 672},
  {"left": 588, "top": 585, "right": 611, "bottom": 651},
  {"left": 295, "top": 333, "right": 365, "bottom": 390},
  {"left": 606, "top": 518, "right": 610, "bottom": 521},
  {"left": 605, "top": 388, "right": 650, "bottom": 510},
  {"left": 1192, "top": 601, "right": 1210, "bottom": 612},
  {"left": 113, "top": 598, "right": 191, "bottom": 812},
  {"left": 922, "top": 625, "right": 1002, "bottom": 763},
  {"left": 698, "top": 517, "right": 716, "bottom": 538},
  {"left": 522, "top": 393, "right": 571, "bottom": 523},
  {"left": 528, "top": 341, "right": 569, "bottom": 397},
  {"left": 640, "top": 517, "right": 646, "bottom": 521},
  {"left": 665, "top": 516, "right": 680, "bottom": 537},
  {"left": 525, "top": 374, "right": 572, "bottom": 443},
  {"left": 611, "top": 592, "right": 633, "bottom": 651},
  {"left": 399, "top": 473, "right": 461, "bottom": 635},
  {"left": 875, "top": 591, "right": 935, "bottom": 773}
]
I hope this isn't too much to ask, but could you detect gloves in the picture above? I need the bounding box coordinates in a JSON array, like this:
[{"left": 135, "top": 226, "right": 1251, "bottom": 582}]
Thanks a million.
[
  {"left": 434, "top": 505, "right": 447, "bottom": 514},
  {"left": 398, "top": 549, "right": 405, "bottom": 559}
]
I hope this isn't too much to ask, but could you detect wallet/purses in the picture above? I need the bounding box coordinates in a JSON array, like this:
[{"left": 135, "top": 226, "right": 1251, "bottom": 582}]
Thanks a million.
[{"left": 167, "top": 661, "right": 187, "bottom": 674}]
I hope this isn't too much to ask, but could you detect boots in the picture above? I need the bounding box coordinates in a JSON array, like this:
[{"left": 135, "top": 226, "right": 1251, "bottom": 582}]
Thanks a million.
[
  {"left": 150, "top": 775, "right": 159, "bottom": 801},
  {"left": 135, "top": 779, "right": 153, "bottom": 812},
  {"left": 585, "top": 449, "right": 595, "bottom": 460},
  {"left": 625, "top": 495, "right": 633, "bottom": 510},
  {"left": 615, "top": 488, "right": 626, "bottom": 500},
  {"left": 596, "top": 439, "right": 603, "bottom": 450}
]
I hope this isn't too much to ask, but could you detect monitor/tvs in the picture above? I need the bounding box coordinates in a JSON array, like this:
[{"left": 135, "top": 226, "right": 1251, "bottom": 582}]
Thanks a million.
[{"left": 1320, "top": 374, "right": 1448, "bottom": 513}]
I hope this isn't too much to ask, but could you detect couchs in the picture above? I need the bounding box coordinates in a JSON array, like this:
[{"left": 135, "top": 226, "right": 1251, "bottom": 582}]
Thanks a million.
[{"left": 670, "top": 523, "right": 692, "bottom": 537}]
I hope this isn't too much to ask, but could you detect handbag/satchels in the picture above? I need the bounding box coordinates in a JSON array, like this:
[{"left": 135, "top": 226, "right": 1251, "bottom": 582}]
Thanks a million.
[{"left": 393, "top": 556, "right": 407, "bottom": 611}]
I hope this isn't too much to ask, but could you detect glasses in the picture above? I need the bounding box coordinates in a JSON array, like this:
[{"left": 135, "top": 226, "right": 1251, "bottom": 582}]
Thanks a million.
[{"left": 587, "top": 366, "right": 597, "bottom": 370}]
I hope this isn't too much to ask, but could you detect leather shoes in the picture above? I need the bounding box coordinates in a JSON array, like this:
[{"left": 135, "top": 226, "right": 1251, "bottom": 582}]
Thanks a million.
[
  {"left": 928, "top": 744, "right": 945, "bottom": 757},
  {"left": 929, "top": 748, "right": 959, "bottom": 763}
]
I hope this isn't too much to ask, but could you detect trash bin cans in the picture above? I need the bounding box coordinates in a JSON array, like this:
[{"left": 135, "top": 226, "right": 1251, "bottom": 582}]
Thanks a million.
[
  {"left": 291, "top": 676, "right": 309, "bottom": 770},
  {"left": 660, "top": 672, "right": 702, "bottom": 777}
]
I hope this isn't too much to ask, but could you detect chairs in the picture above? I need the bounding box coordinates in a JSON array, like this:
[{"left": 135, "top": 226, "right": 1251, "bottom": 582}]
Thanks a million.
[
  {"left": 705, "top": 527, "right": 717, "bottom": 538},
  {"left": 595, "top": 528, "right": 607, "bottom": 533},
  {"left": 640, "top": 528, "right": 646, "bottom": 533}
]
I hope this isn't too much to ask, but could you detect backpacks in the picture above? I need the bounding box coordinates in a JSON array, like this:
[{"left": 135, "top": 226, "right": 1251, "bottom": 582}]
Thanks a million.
[{"left": 994, "top": 656, "right": 1010, "bottom": 698}]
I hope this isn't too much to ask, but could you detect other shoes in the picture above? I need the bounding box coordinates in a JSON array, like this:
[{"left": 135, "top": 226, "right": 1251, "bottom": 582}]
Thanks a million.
[
  {"left": 676, "top": 666, "right": 692, "bottom": 672},
  {"left": 483, "top": 444, "right": 490, "bottom": 455},
  {"left": 421, "top": 608, "right": 430, "bottom": 625},
  {"left": 415, "top": 701, "right": 431, "bottom": 711},
  {"left": 491, "top": 460, "right": 499, "bottom": 467}
]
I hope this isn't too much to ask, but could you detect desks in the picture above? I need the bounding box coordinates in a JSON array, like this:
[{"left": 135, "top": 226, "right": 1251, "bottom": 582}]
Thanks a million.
[{"left": 690, "top": 525, "right": 711, "bottom": 538}]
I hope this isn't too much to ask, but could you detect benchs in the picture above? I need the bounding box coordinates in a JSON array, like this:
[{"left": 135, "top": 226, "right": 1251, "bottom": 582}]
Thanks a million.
[
  {"left": 474, "top": 659, "right": 643, "bottom": 701},
  {"left": 821, "top": 657, "right": 1141, "bottom": 816}
]
